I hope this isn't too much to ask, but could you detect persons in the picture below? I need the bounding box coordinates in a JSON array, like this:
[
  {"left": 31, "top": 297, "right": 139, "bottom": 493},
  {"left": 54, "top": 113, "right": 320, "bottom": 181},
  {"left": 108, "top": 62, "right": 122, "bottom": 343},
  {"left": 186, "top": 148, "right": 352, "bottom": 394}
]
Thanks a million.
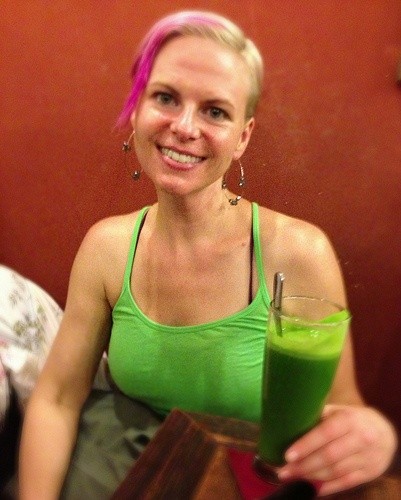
[{"left": 18, "top": 10, "right": 398, "bottom": 500}]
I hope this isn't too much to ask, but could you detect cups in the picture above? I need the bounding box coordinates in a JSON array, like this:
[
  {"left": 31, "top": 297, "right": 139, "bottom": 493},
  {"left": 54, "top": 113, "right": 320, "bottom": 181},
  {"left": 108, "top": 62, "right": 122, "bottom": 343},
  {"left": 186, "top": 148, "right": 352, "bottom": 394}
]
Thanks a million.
[{"left": 253, "top": 295, "right": 351, "bottom": 486}]
